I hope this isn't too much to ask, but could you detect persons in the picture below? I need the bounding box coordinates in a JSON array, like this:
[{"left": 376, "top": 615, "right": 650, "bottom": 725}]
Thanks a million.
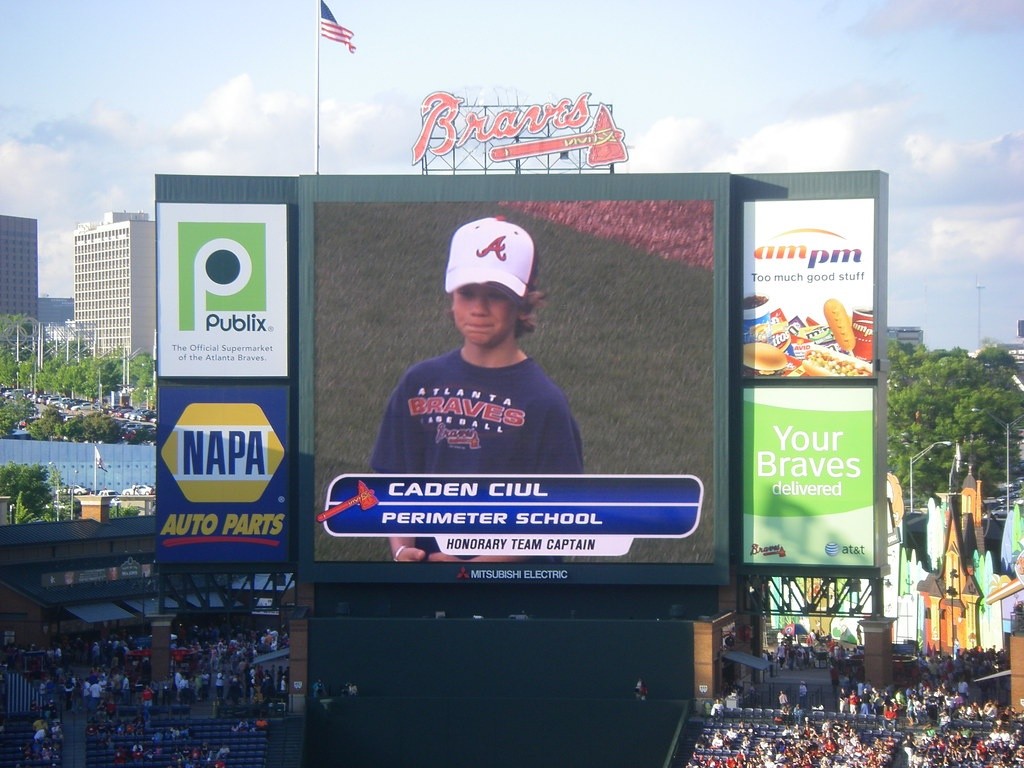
[
  {"left": 684, "top": 621, "right": 1024, "bottom": 768},
  {"left": 311, "top": 677, "right": 327, "bottom": 697},
  {"left": 368, "top": 214, "right": 586, "bottom": 563},
  {"left": 0, "top": 617, "right": 292, "bottom": 768},
  {"left": 633, "top": 675, "right": 649, "bottom": 704},
  {"left": 340, "top": 678, "right": 360, "bottom": 698}
]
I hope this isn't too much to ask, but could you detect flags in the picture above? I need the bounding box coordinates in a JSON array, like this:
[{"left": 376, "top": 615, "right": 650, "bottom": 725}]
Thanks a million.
[{"left": 320, "top": 0, "right": 356, "bottom": 54}]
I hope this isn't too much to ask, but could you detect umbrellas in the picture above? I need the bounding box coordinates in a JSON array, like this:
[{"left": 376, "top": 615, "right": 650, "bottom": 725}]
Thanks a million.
[{"left": 781, "top": 623, "right": 810, "bottom": 637}]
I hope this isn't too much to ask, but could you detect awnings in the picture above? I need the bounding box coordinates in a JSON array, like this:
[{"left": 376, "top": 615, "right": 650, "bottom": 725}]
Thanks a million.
[
  {"left": 723, "top": 649, "right": 773, "bottom": 671},
  {"left": 225, "top": 572, "right": 296, "bottom": 591},
  {"left": 61, "top": 589, "right": 246, "bottom": 625}
]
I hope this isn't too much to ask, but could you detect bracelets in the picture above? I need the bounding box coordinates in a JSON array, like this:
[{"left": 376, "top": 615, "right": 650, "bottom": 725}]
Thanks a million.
[{"left": 393, "top": 544, "right": 406, "bottom": 562}]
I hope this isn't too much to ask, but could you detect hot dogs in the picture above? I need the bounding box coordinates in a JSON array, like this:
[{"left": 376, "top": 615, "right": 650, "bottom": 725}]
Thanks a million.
[{"left": 802, "top": 344, "right": 873, "bottom": 377}]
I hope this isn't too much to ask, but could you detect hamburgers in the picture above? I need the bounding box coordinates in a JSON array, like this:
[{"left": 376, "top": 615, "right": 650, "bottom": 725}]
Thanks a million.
[{"left": 742, "top": 343, "right": 789, "bottom": 378}]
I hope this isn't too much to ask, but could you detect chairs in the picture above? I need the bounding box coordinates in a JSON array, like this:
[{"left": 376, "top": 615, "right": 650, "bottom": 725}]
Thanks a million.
[
  {"left": 683, "top": 707, "right": 1024, "bottom": 767},
  {"left": 0, "top": 713, "right": 277, "bottom": 768}
]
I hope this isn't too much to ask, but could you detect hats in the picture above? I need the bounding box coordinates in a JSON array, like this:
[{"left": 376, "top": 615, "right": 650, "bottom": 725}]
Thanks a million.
[{"left": 446, "top": 214, "right": 536, "bottom": 297}]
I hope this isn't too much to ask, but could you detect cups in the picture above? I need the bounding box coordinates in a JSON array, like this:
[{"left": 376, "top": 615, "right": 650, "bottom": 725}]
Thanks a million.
[
  {"left": 852, "top": 309, "right": 873, "bottom": 361},
  {"left": 744, "top": 298, "right": 770, "bottom": 345}
]
